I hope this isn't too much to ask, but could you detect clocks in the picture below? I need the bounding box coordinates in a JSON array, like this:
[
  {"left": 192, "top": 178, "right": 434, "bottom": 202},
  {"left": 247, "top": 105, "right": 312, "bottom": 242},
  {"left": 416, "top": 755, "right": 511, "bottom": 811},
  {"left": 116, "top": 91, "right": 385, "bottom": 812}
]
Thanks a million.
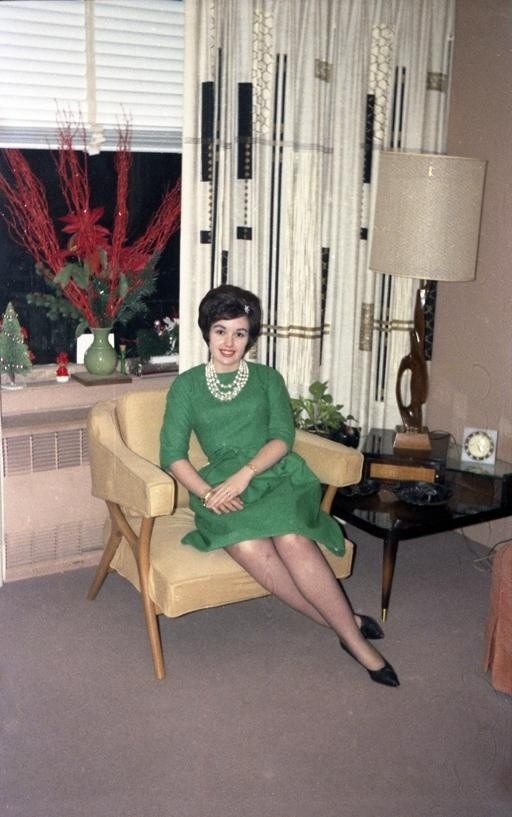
[{"left": 456, "top": 425, "right": 499, "bottom": 466}]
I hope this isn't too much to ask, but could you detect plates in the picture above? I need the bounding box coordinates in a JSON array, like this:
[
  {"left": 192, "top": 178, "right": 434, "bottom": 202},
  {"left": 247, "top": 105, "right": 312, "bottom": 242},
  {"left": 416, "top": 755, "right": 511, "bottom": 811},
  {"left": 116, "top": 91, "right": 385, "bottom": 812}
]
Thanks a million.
[{"left": 399, "top": 486, "right": 454, "bottom": 505}]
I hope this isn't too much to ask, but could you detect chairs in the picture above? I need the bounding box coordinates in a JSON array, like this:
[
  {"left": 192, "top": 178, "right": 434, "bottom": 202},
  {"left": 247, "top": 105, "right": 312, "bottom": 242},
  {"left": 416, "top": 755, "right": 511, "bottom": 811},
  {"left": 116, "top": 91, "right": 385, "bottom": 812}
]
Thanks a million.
[{"left": 85, "top": 386, "right": 361, "bottom": 680}]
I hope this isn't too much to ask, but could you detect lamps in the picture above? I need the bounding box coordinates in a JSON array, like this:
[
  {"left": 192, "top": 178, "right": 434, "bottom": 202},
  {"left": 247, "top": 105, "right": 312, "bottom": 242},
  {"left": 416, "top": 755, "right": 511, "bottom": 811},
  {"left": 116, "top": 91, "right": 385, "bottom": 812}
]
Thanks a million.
[{"left": 364, "top": 149, "right": 487, "bottom": 461}]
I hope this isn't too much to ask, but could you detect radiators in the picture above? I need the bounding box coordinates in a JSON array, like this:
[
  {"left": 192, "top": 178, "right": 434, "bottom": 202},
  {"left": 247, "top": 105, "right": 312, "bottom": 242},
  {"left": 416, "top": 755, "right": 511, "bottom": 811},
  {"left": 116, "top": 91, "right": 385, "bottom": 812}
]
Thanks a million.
[{"left": 2, "top": 420, "right": 118, "bottom": 572}]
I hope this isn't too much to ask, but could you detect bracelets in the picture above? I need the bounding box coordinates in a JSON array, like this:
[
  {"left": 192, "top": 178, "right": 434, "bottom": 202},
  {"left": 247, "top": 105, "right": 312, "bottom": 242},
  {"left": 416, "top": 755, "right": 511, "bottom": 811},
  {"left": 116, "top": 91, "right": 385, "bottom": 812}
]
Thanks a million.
[
  {"left": 201, "top": 490, "right": 212, "bottom": 507},
  {"left": 246, "top": 464, "right": 258, "bottom": 475}
]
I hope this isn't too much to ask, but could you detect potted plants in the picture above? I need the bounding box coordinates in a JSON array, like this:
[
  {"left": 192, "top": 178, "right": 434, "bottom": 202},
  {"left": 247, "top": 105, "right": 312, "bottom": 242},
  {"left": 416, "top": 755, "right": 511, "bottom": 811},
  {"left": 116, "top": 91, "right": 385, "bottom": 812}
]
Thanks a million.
[{"left": 287, "top": 382, "right": 361, "bottom": 449}]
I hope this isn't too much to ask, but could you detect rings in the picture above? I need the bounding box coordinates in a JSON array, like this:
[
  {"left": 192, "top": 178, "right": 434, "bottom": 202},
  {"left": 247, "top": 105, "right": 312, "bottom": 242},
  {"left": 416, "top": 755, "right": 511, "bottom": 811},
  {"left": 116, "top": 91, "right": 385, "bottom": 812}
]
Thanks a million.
[{"left": 227, "top": 493, "right": 230, "bottom": 496}]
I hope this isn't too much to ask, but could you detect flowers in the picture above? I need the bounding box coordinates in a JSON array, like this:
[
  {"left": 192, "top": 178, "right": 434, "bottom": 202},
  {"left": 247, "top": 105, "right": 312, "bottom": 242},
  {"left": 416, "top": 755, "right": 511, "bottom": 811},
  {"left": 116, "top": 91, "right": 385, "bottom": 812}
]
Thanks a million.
[{"left": 4, "top": 114, "right": 182, "bottom": 329}]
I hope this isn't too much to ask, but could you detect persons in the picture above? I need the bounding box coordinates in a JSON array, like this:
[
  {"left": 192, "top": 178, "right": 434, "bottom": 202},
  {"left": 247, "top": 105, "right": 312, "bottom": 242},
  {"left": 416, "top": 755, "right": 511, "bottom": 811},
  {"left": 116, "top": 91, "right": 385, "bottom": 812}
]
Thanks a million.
[{"left": 160, "top": 284, "right": 401, "bottom": 688}]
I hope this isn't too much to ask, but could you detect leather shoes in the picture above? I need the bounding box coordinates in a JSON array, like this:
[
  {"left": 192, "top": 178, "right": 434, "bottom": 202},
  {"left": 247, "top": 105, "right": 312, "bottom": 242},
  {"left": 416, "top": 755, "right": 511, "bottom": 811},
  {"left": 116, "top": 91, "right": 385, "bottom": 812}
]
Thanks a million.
[
  {"left": 353, "top": 609, "right": 386, "bottom": 640},
  {"left": 340, "top": 638, "right": 401, "bottom": 688}
]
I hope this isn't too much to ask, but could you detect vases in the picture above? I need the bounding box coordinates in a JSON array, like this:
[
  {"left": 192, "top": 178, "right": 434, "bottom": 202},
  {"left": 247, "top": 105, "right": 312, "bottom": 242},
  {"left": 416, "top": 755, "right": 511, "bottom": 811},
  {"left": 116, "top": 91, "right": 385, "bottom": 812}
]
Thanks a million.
[{"left": 84, "top": 326, "right": 117, "bottom": 376}]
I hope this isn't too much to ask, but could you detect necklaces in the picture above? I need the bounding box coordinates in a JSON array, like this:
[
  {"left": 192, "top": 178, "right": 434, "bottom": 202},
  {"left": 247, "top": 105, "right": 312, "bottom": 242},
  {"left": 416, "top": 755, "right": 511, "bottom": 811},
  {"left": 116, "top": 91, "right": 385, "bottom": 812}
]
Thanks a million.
[{"left": 205, "top": 357, "right": 249, "bottom": 401}]
